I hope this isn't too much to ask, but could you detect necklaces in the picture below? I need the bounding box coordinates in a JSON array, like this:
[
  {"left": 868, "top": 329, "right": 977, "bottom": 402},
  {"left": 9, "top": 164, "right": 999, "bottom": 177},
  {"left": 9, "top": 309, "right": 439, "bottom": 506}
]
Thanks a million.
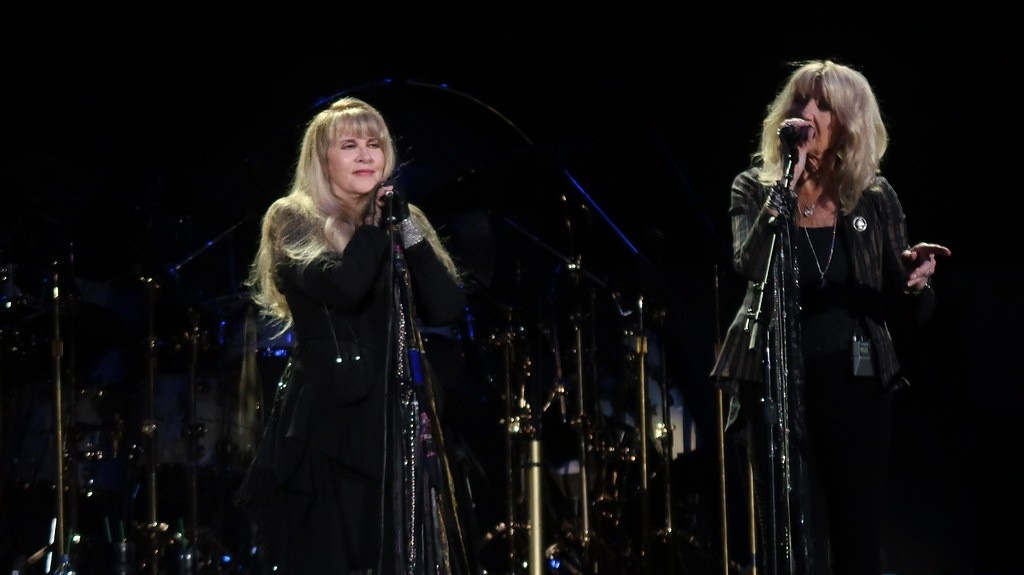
[{"left": 795, "top": 197, "right": 838, "bottom": 291}]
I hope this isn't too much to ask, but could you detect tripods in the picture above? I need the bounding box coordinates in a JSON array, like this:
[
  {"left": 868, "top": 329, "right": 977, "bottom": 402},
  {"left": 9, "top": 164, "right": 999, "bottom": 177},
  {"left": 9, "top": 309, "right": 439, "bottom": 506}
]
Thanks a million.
[
  {"left": 475, "top": 265, "right": 745, "bottom": 575},
  {"left": 13, "top": 284, "right": 248, "bottom": 575}
]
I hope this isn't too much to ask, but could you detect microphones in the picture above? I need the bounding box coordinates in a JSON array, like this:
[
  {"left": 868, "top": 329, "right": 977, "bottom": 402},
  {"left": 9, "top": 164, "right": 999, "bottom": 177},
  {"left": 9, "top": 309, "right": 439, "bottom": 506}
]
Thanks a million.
[
  {"left": 385, "top": 190, "right": 394, "bottom": 198},
  {"left": 779, "top": 125, "right": 801, "bottom": 143}
]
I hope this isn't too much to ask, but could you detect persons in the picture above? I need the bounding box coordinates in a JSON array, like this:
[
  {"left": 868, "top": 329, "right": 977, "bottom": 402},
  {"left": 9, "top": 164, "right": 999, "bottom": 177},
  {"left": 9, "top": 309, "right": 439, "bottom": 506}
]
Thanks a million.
[
  {"left": 731, "top": 59, "right": 950, "bottom": 575},
  {"left": 243, "top": 96, "right": 462, "bottom": 575}
]
